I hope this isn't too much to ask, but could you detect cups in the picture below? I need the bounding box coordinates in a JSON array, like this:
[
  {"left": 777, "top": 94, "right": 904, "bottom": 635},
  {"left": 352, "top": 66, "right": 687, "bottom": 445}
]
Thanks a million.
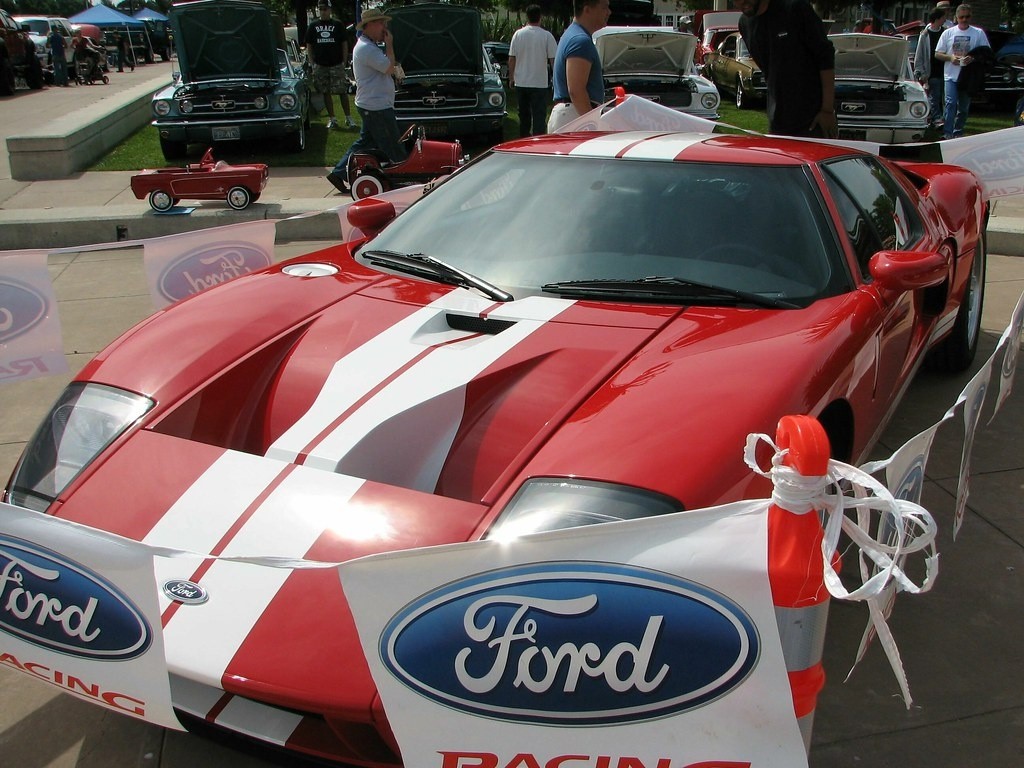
[{"left": 960, "top": 58, "right": 968, "bottom": 66}]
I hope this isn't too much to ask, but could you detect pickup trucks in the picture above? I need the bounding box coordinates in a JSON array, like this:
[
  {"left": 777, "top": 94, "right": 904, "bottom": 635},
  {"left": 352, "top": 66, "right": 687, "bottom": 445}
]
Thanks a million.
[{"left": 101, "top": 21, "right": 172, "bottom": 63}]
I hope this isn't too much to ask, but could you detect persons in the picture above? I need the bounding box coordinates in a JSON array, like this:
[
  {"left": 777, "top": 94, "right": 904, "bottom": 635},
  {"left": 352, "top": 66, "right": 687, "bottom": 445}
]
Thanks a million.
[
  {"left": 508, "top": 6, "right": 557, "bottom": 138},
  {"left": 304, "top": 0, "right": 357, "bottom": 129},
  {"left": 851, "top": 20, "right": 883, "bottom": 34},
  {"left": 914, "top": 1, "right": 992, "bottom": 140},
  {"left": 326, "top": 8, "right": 408, "bottom": 194},
  {"left": 674, "top": 16, "right": 702, "bottom": 53},
  {"left": 0, "top": 25, "right": 151, "bottom": 96},
  {"left": 547, "top": 0, "right": 611, "bottom": 134},
  {"left": 738, "top": 0, "right": 838, "bottom": 139}
]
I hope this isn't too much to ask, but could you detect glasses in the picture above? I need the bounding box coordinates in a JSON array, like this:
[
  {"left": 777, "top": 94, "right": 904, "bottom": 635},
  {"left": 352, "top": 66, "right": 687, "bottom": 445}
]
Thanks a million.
[{"left": 958, "top": 15, "right": 970, "bottom": 19}]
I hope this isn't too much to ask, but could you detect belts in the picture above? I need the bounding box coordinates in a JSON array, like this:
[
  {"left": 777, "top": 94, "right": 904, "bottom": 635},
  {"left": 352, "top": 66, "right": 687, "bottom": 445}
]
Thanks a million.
[{"left": 553, "top": 98, "right": 598, "bottom": 109}]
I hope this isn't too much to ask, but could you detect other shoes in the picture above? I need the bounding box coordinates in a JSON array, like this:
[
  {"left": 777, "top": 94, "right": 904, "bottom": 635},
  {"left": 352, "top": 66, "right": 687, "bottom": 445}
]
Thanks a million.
[
  {"left": 327, "top": 118, "right": 337, "bottom": 130},
  {"left": 940, "top": 133, "right": 951, "bottom": 140},
  {"left": 953, "top": 127, "right": 962, "bottom": 135},
  {"left": 345, "top": 120, "right": 357, "bottom": 128},
  {"left": 326, "top": 171, "right": 349, "bottom": 194},
  {"left": 933, "top": 119, "right": 944, "bottom": 129}
]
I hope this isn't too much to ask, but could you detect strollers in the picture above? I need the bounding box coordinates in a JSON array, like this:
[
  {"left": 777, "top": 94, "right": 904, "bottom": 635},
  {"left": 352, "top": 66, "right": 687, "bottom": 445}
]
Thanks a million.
[{"left": 75, "top": 47, "right": 109, "bottom": 85}]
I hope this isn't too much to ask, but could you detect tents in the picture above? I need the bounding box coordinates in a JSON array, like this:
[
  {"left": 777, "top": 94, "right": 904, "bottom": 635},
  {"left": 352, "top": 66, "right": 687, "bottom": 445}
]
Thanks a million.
[{"left": 67, "top": 4, "right": 170, "bottom": 67}]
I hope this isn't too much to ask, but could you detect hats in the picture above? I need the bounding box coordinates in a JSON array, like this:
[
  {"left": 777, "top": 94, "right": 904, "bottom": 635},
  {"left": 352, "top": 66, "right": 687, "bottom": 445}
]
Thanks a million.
[
  {"left": 356, "top": 8, "right": 392, "bottom": 30},
  {"left": 318, "top": 0, "right": 331, "bottom": 8},
  {"left": 934, "top": 1, "right": 952, "bottom": 9}
]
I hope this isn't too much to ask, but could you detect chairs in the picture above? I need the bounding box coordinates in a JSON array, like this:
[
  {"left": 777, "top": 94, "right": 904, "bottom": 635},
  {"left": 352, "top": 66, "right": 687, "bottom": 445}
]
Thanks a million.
[{"left": 353, "top": 148, "right": 391, "bottom": 169}]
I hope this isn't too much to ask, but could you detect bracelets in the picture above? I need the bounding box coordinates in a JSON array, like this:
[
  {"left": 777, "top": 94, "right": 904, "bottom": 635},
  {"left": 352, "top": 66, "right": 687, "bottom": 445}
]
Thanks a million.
[{"left": 821, "top": 109, "right": 836, "bottom": 116}]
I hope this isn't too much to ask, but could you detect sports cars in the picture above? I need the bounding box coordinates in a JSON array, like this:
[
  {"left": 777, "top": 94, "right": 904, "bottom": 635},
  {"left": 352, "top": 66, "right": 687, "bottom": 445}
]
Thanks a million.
[{"left": 0, "top": 129, "right": 989, "bottom": 768}]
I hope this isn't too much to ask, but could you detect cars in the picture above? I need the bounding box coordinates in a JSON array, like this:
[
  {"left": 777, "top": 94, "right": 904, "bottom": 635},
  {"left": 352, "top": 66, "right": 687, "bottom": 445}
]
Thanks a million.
[
  {"left": 345, "top": 123, "right": 470, "bottom": 202},
  {"left": 853, "top": 18, "right": 1024, "bottom": 113},
  {"left": 607, "top": 0, "right": 662, "bottom": 25},
  {"left": 702, "top": 32, "right": 768, "bottom": 110},
  {"left": 484, "top": 41, "right": 552, "bottom": 90},
  {"left": 12, "top": 15, "right": 77, "bottom": 82},
  {"left": 827, "top": 33, "right": 930, "bottom": 144},
  {"left": 270, "top": 12, "right": 312, "bottom": 75},
  {"left": 130, "top": 147, "right": 269, "bottom": 210},
  {"left": 591, "top": 25, "right": 720, "bottom": 121},
  {"left": 377, "top": 2, "right": 506, "bottom": 151},
  {"left": 692, "top": 10, "right": 743, "bottom": 64},
  {"left": 0, "top": 9, "right": 43, "bottom": 95},
  {"left": 150, "top": 0, "right": 311, "bottom": 162}
]
